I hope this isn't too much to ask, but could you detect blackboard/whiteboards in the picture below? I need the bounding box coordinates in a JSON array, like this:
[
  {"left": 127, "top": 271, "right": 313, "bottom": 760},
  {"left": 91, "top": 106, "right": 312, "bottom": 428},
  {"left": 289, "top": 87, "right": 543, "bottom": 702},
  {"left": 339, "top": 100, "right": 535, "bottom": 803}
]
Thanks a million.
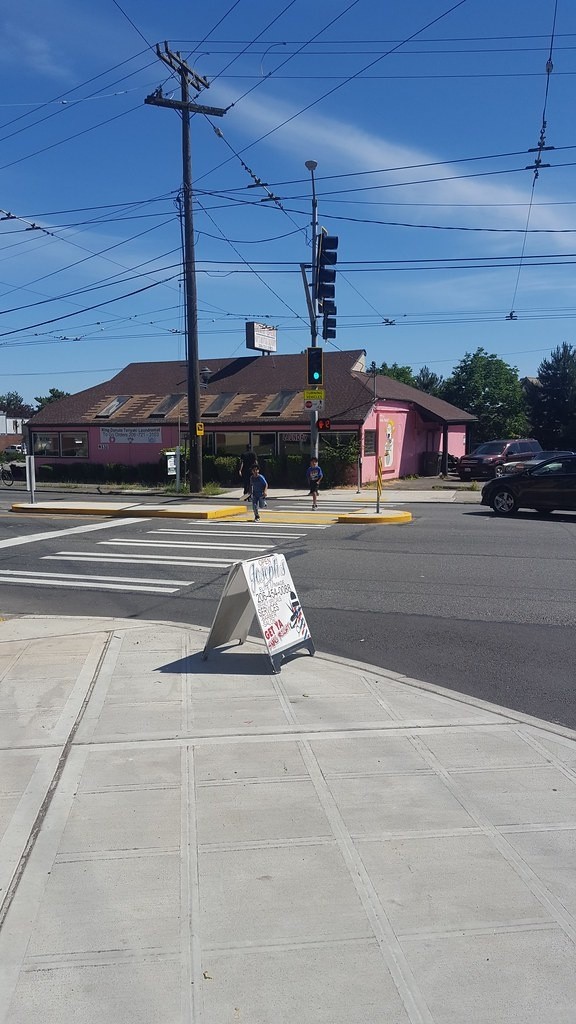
[{"left": 206, "top": 553, "right": 312, "bottom": 656}]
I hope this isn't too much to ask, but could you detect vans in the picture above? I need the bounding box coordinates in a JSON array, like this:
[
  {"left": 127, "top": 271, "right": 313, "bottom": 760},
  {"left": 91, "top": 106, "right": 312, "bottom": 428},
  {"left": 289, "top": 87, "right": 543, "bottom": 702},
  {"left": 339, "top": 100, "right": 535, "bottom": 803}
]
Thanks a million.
[{"left": 3, "top": 444, "right": 27, "bottom": 455}]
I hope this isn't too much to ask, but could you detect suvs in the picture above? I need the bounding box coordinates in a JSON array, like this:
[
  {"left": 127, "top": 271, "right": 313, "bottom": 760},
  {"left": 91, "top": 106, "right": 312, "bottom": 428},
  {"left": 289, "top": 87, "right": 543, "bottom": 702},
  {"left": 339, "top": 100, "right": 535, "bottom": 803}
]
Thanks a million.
[{"left": 454, "top": 438, "right": 543, "bottom": 481}]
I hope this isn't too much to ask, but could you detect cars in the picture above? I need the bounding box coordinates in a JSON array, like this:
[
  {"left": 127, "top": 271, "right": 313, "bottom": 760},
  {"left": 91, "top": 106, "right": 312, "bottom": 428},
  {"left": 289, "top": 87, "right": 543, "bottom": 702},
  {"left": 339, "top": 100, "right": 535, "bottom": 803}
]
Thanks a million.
[
  {"left": 502, "top": 449, "right": 575, "bottom": 475},
  {"left": 480, "top": 454, "right": 576, "bottom": 516}
]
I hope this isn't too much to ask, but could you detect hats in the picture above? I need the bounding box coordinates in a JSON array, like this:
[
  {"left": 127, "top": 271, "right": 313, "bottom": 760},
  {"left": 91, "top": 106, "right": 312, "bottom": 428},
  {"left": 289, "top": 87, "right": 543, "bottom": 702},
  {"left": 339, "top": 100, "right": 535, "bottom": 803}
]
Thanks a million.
[{"left": 251, "top": 464, "right": 260, "bottom": 470}]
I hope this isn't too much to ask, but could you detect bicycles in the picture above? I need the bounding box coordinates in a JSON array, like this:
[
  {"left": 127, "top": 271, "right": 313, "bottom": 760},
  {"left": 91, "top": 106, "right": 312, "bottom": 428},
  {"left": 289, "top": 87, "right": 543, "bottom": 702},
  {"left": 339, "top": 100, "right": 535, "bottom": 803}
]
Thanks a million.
[{"left": 0, "top": 462, "right": 15, "bottom": 487}]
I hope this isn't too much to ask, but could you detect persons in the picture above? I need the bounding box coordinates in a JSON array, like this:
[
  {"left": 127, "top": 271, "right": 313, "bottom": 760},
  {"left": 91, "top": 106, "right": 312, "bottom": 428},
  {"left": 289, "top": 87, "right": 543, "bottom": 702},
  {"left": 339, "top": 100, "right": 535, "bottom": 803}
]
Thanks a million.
[
  {"left": 247, "top": 465, "right": 268, "bottom": 521},
  {"left": 306, "top": 458, "right": 323, "bottom": 511},
  {"left": 239, "top": 444, "right": 259, "bottom": 501}
]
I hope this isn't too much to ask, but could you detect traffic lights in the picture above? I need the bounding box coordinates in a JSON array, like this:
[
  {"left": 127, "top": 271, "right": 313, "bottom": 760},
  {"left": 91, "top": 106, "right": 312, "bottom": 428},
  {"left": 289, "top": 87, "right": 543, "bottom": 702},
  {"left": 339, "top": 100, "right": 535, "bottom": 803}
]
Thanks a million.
[
  {"left": 314, "top": 226, "right": 339, "bottom": 299},
  {"left": 305, "top": 347, "right": 324, "bottom": 387},
  {"left": 317, "top": 417, "right": 332, "bottom": 431},
  {"left": 322, "top": 300, "right": 338, "bottom": 340}
]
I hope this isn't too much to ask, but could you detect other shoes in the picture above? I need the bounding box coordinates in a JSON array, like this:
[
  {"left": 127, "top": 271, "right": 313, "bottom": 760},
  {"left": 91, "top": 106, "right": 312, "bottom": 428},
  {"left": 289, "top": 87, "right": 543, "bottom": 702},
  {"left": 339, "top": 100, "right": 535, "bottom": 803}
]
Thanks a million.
[
  {"left": 264, "top": 502, "right": 267, "bottom": 509},
  {"left": 312, "top": 505, "right": 318, "bottom": 510},
  {"left": 254, "top": 518, "right": 259, "bottom": 522}
]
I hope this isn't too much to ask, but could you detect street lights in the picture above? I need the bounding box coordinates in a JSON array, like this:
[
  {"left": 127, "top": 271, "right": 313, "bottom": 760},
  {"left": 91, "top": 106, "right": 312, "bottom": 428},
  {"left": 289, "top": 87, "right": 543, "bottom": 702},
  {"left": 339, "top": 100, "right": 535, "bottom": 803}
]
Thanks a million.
[{"left": 301, "top": 159, "right": 322, "bottom": 466}]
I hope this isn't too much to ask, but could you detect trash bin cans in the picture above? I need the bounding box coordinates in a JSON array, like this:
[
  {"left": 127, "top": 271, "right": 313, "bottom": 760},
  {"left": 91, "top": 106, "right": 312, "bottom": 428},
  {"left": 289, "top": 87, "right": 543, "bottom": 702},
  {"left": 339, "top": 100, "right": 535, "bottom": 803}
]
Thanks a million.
[{"left": 423, "top": 451, "right": 443, "bottom": 476}]
